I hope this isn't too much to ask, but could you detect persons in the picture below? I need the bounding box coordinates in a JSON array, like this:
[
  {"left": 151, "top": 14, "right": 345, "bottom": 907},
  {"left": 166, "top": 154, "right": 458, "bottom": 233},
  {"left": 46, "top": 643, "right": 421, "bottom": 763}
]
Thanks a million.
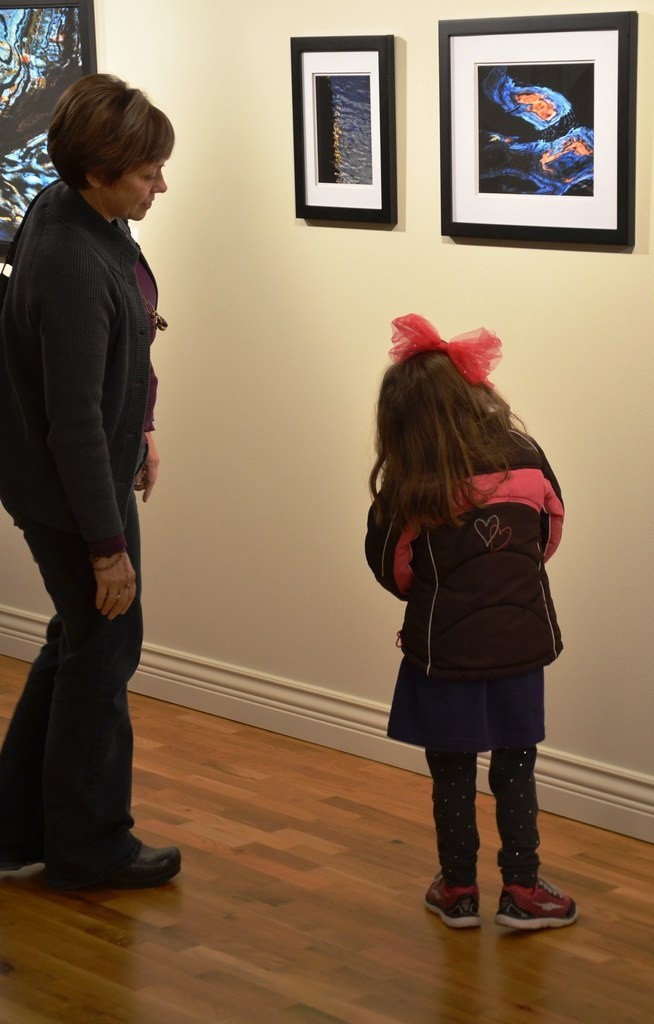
[
  {"left": 0, "top": 72, "right": 184, "bottom": 894},
  {"left": 365, "top": 313, "right": 579, "bottom": 930}
]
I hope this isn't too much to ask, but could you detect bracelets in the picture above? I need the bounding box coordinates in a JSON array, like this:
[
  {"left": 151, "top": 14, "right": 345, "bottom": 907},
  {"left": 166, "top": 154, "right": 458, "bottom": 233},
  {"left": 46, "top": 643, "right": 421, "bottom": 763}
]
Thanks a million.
[{"left": 91, "top": 549, "right": 128, "bottom": 572}]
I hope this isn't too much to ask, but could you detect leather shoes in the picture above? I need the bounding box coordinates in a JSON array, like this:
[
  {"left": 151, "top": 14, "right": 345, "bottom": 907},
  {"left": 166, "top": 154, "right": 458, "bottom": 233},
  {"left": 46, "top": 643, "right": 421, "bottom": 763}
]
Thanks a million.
[
  {"left": 46, "top": 840, "right": 181, "bottom": 890},
  {"left": 0, "top": 845, "right": 54, "bottom": 871}
]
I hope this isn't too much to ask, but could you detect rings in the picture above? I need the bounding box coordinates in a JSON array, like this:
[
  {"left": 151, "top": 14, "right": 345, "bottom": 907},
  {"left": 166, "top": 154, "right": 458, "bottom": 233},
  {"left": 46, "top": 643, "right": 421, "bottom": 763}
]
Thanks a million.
[
  {"left": 113, "top": 593, "right": 123, "bottom": 599},
  {"left": 124, "top": 584, "right": 132, "bottom": 588}
]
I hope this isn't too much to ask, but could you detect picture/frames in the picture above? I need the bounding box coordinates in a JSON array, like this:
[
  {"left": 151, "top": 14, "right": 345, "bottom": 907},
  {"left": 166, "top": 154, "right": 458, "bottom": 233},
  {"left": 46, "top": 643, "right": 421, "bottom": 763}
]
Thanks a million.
[
  {"left": 438, "top": 11, "right": 638, "bottom": 246},
  {"left": 290, "top": 34, "right": 398, "bottom": 224},
  {"left": 0, "top": 0, "right": 95, "bottom": 263}
]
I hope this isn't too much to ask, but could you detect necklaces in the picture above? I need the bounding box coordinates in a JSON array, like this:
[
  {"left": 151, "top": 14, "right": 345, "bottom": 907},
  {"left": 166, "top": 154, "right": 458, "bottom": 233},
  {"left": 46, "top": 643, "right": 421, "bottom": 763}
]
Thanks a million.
[{"left": 143, "top": 295, "right": 168, "bottom": 331}]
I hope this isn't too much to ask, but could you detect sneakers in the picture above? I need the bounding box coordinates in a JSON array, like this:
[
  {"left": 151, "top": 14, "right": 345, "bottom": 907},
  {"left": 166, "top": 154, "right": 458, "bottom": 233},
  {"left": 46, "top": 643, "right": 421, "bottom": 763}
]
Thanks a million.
[
  {"left": 425, "top": 872, "right": 481, "bottom": 928},
  {"left": 495, "top": 877, "right": 578, "bottom": 929}
]
